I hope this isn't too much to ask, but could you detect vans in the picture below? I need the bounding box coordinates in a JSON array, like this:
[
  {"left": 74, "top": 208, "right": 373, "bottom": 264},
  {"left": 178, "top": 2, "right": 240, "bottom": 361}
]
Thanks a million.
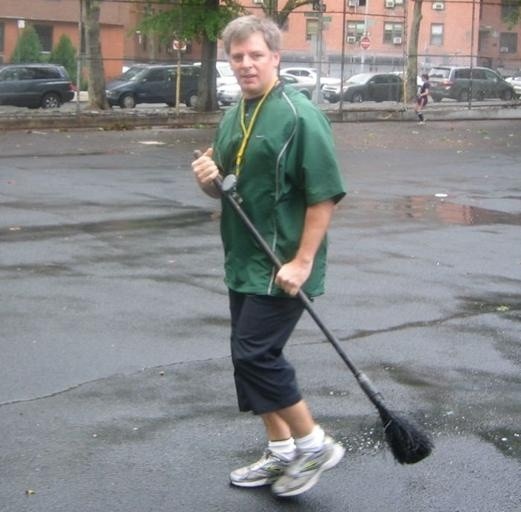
[{"left": 0, "top": 63, "right": 75, "bottom": 109}]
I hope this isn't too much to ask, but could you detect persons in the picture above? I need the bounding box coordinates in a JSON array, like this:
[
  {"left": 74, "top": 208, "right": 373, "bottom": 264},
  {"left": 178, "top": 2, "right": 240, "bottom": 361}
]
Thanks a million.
[
  {"left": 189, "top": 15, "right": 348, "bottom": 500},
  {"left": 414, "top": 73, "right": 433, "bottom": 126}
]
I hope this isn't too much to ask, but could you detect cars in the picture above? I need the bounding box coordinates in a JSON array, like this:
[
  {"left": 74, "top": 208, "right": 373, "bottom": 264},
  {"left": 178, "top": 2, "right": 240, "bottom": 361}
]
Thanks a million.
[
  {"left": 279, "top": 67, "right": 341, "bottom": 88},
  {"left": 322, "top": 73, "right": 404, "bottom": 104},
  {"left": 217, "top": 73, "right": 316, "bottom": 105},
  {"left": 194, "top": 63, "right": 237, "bottom": 84},
  {"left": 428, "top": 66, "right": 521, "bottom": 102}
]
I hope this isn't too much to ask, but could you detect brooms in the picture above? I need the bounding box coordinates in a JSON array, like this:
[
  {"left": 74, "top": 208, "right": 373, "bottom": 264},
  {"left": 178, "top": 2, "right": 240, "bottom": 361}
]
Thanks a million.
[{"left": 192, "top": 147, "right": 436, "bottom": 463}]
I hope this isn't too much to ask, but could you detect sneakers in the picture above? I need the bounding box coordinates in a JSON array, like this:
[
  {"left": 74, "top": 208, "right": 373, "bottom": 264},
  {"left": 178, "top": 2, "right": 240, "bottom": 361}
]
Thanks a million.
[{"left": 230, "top": 432, "right": 346, "bottom": 497}]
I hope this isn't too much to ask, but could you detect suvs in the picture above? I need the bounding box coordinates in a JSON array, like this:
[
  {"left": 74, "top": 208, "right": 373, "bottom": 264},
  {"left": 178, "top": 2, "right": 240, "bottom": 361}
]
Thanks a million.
[{"left": 101, "top": 64, "right": 205, "bottom": 111}]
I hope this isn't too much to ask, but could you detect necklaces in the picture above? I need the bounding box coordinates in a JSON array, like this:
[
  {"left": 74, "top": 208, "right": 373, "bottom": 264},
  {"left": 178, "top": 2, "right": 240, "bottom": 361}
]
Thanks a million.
[{"left": 236, "top": 81, "right": 277, "bottom": 186}]
